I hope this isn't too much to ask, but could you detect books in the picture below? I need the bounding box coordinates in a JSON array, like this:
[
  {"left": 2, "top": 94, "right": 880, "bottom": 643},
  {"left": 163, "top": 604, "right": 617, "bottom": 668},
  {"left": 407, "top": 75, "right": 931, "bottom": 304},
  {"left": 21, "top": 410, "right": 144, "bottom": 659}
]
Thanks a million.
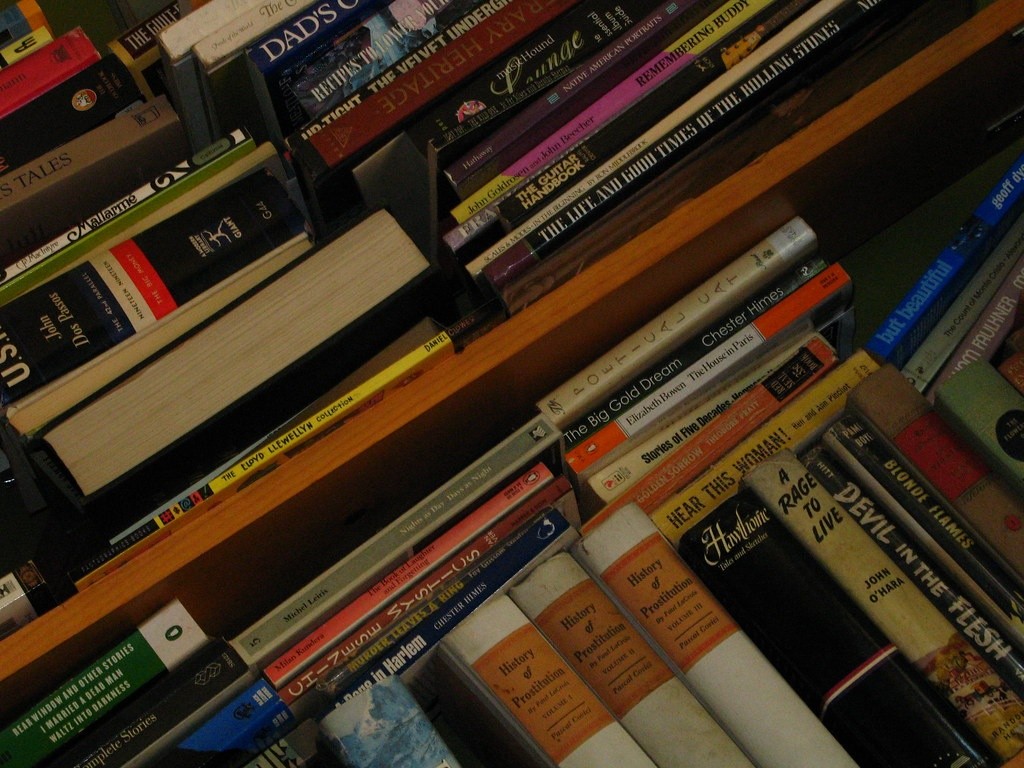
[
  {"left": 229, "top": 415, "right": 568, "bottom": 678},
  {"left": 439, "top": 597, "right": 655, "bottom": 768},
  {"left": 573, "top": 500, "right": 860, "bottom": 768},
  {"left": 0, "top": 0, "right": 1024, "bottom": 768},
  {"left": 508, "top": 552, "right": 753, "bottom": 768},
  {"left": 321, "top": 675, "right": 457, "bottom": 768}
]
